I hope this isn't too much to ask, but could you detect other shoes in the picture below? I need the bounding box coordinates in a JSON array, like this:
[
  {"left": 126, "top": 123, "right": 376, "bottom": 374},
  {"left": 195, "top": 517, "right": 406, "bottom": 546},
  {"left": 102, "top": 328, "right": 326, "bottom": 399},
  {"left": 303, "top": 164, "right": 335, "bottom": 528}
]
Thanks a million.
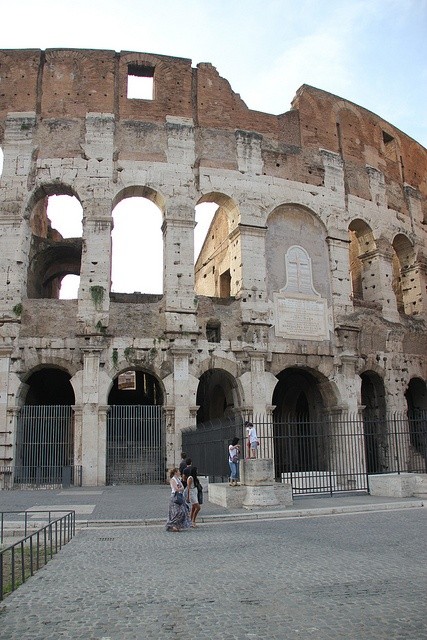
[
  {"left": 235, "top": 484, "right": 240, "bottom": 486},
  {"left": 230, "top": 483, "right": 235, "bottom": 487},
  {"left": 250, "top": 457, "right": 256, "bottom": 459}
]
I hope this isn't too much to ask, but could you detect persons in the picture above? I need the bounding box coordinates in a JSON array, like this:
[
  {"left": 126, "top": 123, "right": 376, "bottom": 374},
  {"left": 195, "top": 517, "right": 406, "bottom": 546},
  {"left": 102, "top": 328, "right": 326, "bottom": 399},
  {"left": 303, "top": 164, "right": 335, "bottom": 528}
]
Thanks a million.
[
  {"left": 179, "top": 452, "right": 198, "bottom": 489},
  {"left": 186, "top": 466, "right": 201, "bottom": 528},
  {"left": 164, "top": 468, "right": 185, "bottom": 532},
  {"left": 228, "top": 437, "right": 241, "bottom": 487},
  {"left": 243, "top": 421, "right": 257, "bottom": 459}
]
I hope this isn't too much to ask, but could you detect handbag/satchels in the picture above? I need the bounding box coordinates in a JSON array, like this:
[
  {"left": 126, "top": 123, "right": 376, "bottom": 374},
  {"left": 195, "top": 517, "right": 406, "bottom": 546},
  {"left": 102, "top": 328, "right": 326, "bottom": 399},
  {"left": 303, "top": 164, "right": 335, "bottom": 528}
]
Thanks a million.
[
  {"left": 174, "top": 492, "right": 183, "bottom": 504},
  {"left": 198, "top": 493, "right": 203, "bottom": 504},
  {"left": 232, "top": 454, "right": 240, "bottom": 464}
]
